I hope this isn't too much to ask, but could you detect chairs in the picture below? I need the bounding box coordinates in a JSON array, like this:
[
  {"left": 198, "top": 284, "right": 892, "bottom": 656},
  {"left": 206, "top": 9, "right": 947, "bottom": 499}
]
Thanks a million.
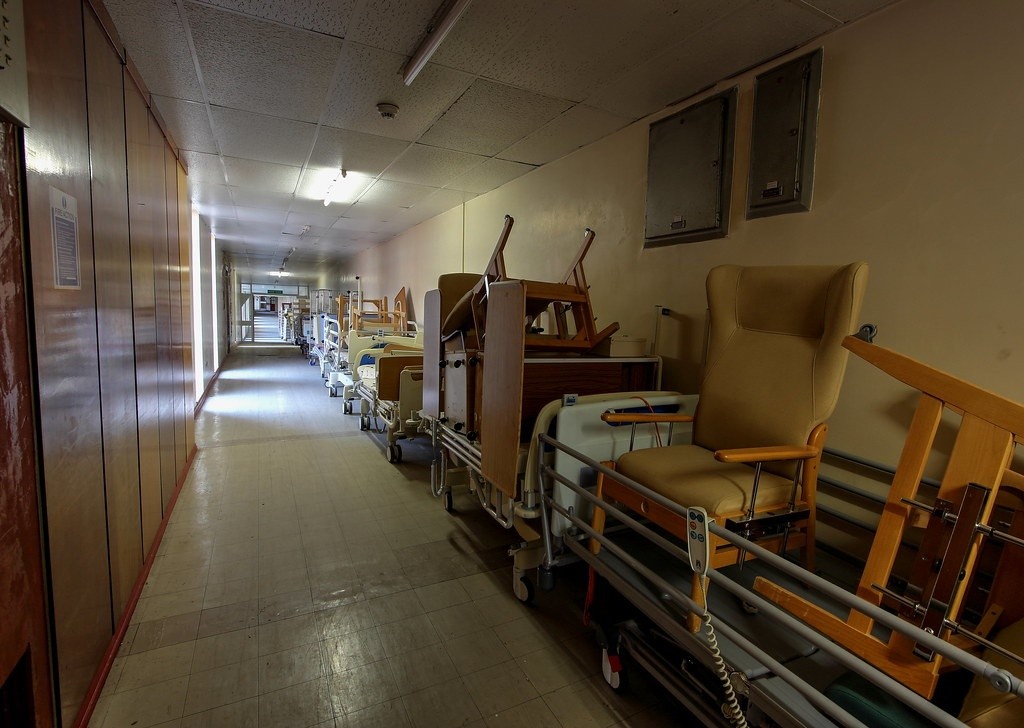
[
  {"left": 584, "top": 257, "right": 868, "bottom": 637},
  {"left": 337, "top": 214, "right": 684, "bottom": 517}
]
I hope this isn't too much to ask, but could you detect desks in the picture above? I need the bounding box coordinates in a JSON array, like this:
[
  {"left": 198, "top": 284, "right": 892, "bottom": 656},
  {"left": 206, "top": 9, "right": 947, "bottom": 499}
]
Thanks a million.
[{"left": 753, "top": 335, "right": 1022, "bottom": 698}]
